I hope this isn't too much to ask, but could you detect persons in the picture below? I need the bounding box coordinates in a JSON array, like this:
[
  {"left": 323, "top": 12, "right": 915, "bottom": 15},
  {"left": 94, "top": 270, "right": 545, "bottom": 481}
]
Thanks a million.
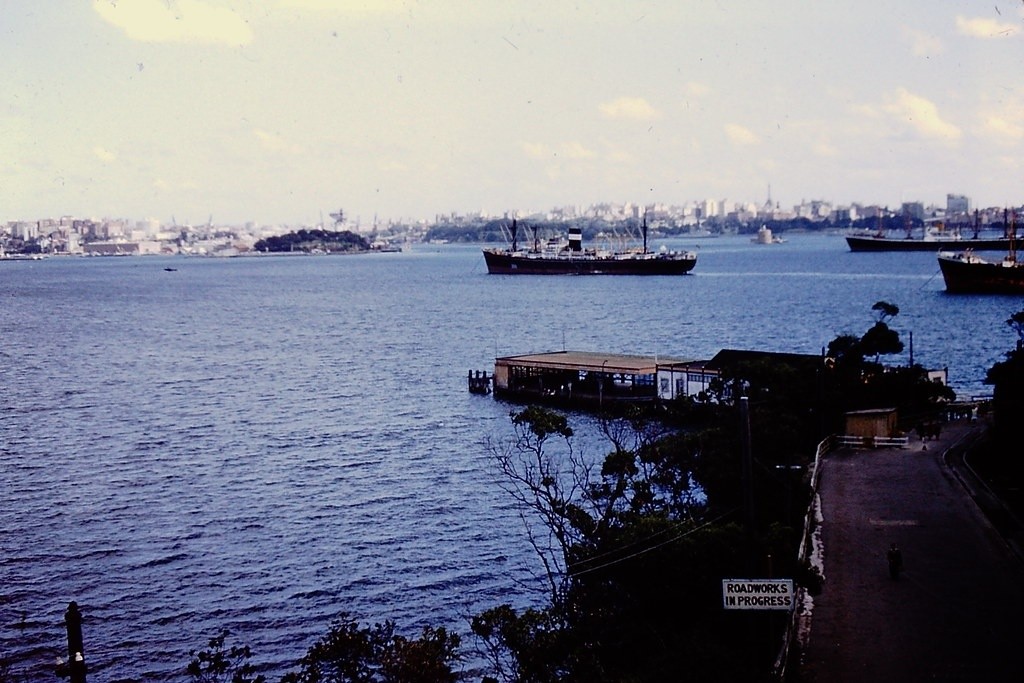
[
  {"left": 887, "top": 543, "right": 904, "bottom": 582},
  {"left": 916, "top": 419, "right": 942, "bottom": 441},
  {"left": 966, "top": 406, "right": 972, "bottom": 423}
]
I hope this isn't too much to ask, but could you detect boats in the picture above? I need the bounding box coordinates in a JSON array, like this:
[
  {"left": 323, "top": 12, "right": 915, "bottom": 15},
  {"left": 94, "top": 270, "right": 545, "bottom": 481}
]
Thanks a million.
[
  {"left": 751, "top": 238, "right": 788, "bottom": 243},
  {"left": 637, "top": 230, "right": 669, "bottom": 239},
  {"left": 672, "top": 226, "right": 720, "bottom": 239},
  {"left": 103, "top": 251, "right": 111, "bottom": 255},
  {"left": 429, "top": 239, "right": 448, "bottom": 245},
  {"left": 482, "top": 218, "right": 698, "bottom": 276},
  {"left": 937, "top": 247, "right": 1024, "bottom": 297},
  {"left": 924, "top": 217, "right": 963, "bottom": 241},
  {"left": 380, "top": 247, "right": 402, "bottom": 252},
  {"left": 113, "top": 252, "right": 127, "bottom": 256},
  {"left": 970, "top": 235, "right": 980, "bottom": 239},
  {"left": 123, "top": 252, "right": 133, "bottom": 255},
  {"left": 904, "top": 236, "right": 914, "bottom": 239},
  {"left": 871, "top": 234, "right": 886, "bottom": 239},
  {"left": 164, "top": 268, "right": 178, "bottom": 271},
  {"left": 845, "top": 234, "right": 1024, "bottom": 252}
]
